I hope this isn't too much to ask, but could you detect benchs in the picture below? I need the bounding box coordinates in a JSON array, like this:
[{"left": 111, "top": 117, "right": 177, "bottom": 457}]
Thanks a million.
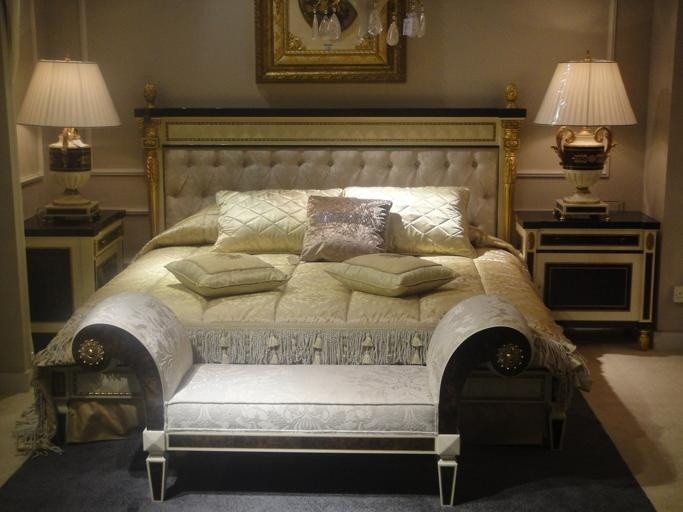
[{"left": 72, "top": 293, "right": 534, "bottom": 508}]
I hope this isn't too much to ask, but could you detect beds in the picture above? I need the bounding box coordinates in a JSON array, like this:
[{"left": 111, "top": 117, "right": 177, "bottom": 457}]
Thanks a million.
[{"left": 12, "top": 80, "right": 595, "bottom": 459}]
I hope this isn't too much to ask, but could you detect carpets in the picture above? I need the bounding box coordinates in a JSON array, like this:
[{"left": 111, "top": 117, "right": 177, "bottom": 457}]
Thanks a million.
[{"left": 0, "top": 386, "right": 659, "bottom": 512}]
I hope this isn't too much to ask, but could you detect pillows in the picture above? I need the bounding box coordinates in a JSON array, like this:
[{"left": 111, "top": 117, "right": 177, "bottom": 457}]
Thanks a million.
[
  {"left": 212, "top": 187, "right": 342, "bottom": 255},
  {"left": 300, "top": 195, "right": 392, "bottom": 260},
  {"left": 325, "top": 251, "right": 461, "bottom": 300},
  {"left": 343, "top": 186, "right": 476, "bottom": 257},
  {"left": 164, "top": 252, "right": 292, "bottom": 301}
]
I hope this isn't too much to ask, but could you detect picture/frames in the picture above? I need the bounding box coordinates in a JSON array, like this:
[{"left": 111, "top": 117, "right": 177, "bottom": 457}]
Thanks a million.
[{"left": 253, "top": 0, "right": 408, "bottom": 84}]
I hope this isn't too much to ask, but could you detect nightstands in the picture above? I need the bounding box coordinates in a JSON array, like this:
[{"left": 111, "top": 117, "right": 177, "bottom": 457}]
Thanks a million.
[
  {"left": 24, "top": 206, "right": 127, "bottom": 353},
  {"left": 513, "top": 208, "right": 662, "bottom": 351}
]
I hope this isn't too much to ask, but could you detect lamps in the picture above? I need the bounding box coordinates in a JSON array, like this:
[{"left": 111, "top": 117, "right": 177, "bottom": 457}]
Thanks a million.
[
  {"left": 532, "top": 50, "right": 639, "bottom": 221},
  {"left": 17, "top": 54, "right": 123, "bottom": 225}
]
